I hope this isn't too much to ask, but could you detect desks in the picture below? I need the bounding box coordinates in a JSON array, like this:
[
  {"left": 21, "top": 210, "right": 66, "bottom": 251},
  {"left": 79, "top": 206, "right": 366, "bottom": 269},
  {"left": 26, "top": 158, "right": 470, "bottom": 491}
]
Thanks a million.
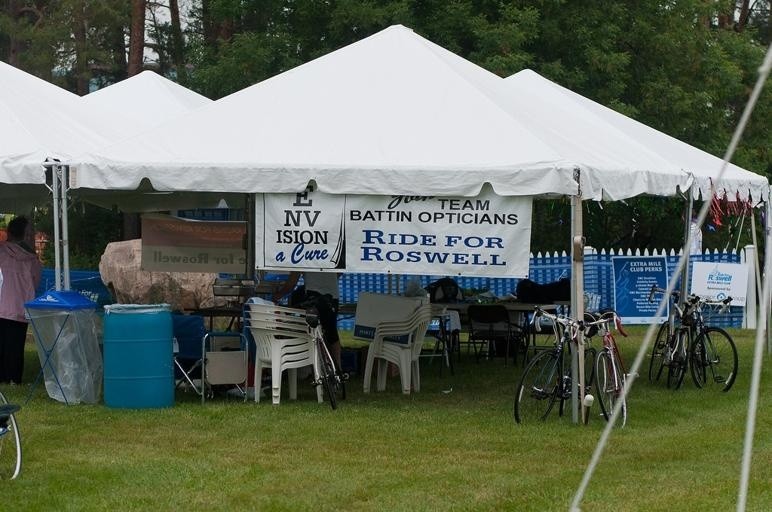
[{"left": 430, "top": 303, "right": 570, "bottom": 368}]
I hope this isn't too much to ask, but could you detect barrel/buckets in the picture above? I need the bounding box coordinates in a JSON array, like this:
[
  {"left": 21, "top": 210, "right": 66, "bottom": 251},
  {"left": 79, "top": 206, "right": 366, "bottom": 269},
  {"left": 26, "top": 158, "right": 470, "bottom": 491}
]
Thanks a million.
[{"left": 103, "top": 304, "right": 174, "bottom": 407}]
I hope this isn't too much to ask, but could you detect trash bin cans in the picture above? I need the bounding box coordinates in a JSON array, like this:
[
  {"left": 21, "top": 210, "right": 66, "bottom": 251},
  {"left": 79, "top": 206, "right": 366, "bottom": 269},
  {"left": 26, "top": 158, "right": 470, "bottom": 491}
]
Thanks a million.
[{"left": 101, "top": 304, "right": 175, "bottom": 408}]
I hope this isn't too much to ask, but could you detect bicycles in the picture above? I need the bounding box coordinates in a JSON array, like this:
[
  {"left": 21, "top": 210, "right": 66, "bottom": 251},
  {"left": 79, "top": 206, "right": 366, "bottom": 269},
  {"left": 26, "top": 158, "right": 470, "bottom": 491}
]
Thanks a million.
[
  {"left": 299, "top": 293, "right": 351, "bottom": 410},
  {"left": 647, "top": 282, "right": 739, "bottom": 393},
  {"left": 513, "top": 303, "right": 641, "bottom": 430}
]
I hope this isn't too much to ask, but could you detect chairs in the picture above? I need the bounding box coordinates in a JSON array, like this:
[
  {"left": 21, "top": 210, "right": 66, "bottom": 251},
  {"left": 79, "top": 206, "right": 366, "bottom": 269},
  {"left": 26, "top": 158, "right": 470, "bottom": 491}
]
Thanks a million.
[
  {"left": 175, "top": 302, "right": 324, "bottom": 406},
  {"left": 363, "top": 303, "right": 432, "bottom": 397}
]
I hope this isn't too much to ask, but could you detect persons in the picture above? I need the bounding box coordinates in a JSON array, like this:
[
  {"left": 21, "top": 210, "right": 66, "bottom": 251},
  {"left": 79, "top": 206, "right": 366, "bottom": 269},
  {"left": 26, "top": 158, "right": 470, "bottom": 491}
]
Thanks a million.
[
  {"left": 271, "top": 272, "right": 349, "bottom": 381},
  {"left": 0, "top": 215, "right": 42, "bottom": 386}
]
